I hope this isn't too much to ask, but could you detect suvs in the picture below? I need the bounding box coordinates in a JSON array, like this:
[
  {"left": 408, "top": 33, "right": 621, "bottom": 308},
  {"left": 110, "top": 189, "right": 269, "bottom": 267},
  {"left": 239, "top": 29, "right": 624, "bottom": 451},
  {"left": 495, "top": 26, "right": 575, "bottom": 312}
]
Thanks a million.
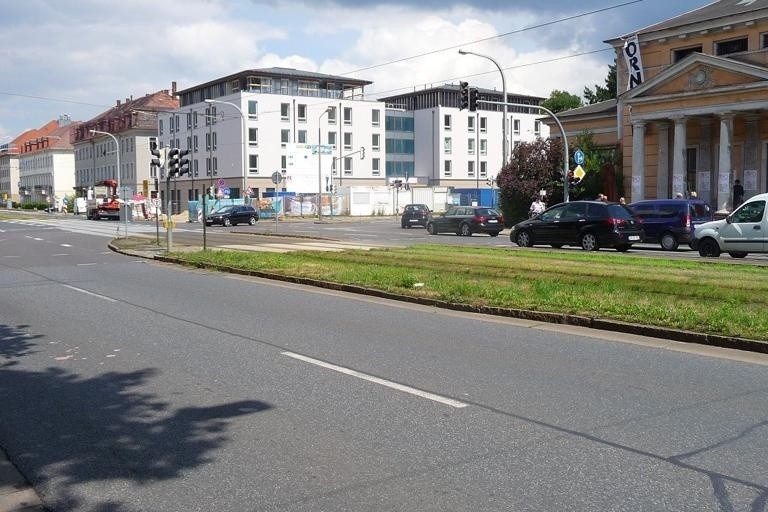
[{"left": 203, "top": 204, "right": 260, "bottom": 228}]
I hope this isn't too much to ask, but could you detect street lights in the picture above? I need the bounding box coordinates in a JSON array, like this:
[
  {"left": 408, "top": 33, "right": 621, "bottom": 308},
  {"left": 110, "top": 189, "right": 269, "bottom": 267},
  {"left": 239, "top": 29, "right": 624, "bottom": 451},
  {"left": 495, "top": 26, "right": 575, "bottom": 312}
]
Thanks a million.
[
  {"left": 317, "top": 107, "right": 333, "bottom": 220},
  {"left": 204, "top": 98, "right": 248, "bottom": 203},
  {"left": 89, "top": 129, "right": 121, "bottom": 196},
  {"left": 458, "top": 49, "right": 508, "bottom": 172}
]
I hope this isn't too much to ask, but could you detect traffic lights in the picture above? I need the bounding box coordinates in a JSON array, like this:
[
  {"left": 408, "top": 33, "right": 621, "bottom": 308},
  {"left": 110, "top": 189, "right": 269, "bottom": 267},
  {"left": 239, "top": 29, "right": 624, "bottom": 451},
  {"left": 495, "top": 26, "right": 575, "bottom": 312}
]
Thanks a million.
[
  {"left": 568, "top": 170, "right": 574, "bottom": 190},
  {"left": 470, "top": 88, "right": 479, "bottom": 111},
  {"left": 151, "top": 148, "right": 164, "bottom": 168},
  {"left": 398, "top": 180, "right": 402, "bottom": 187},
  {"left": 361, "top": 147, "right": 365, "bottom": 160},
  {"left": 459, "top": 82, "right": 469, "bottom": 111},
  {"left": 164, "top": 147, "right": 180, "bottom": 179},
  {"left": 486, "top": 177, "right": 492, "bottom": 186},
  {"left": 177, "top": 149, "right": 189, "bottom": 176}
]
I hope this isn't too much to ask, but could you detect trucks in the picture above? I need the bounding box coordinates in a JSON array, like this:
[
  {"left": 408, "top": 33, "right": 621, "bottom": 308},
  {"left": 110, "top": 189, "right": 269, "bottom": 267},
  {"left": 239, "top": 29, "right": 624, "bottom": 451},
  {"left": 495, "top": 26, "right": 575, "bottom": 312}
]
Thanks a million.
[{"left": 87, "top": 188, "right": 121, "bottom": 220}]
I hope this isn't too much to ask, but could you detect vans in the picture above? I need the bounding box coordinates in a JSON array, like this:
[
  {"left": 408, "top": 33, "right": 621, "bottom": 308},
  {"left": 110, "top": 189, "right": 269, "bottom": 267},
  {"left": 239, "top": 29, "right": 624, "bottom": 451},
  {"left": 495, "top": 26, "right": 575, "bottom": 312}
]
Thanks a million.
[{"left": 72, "top": 198, "right": 87, "bottom": 215}]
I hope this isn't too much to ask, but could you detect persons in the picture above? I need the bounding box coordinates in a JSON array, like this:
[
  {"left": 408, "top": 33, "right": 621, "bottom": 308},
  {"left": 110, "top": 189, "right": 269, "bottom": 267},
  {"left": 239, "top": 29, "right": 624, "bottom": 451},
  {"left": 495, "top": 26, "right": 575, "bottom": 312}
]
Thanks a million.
[
  {"left": 733, "top": 179, "right": 744, "bottom": 211},
  {"left": 690, "top": 191, "right": 697, "bottom": 198},
  {"left": 674, "top": 193, "right": 683, "bottom": 199},
  {"left": 528, "top": 196, "right": 545, "bottom": 218},
  {"left": 602, "top": 196, "right": 608, "bottom": 201},
  {"left": 595, "top": 193, "right": 604, "bottom": 201},
  {"left": 620, "top": 197, "right": 626, "bottom": 205}
]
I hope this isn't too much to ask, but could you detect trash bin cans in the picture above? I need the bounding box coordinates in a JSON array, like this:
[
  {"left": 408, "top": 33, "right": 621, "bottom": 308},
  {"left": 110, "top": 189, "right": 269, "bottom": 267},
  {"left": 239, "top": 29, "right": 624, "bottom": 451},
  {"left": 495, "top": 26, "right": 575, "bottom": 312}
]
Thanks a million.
[{"left": 6, "top": 199, "right": 12, "bottom": 209}]
